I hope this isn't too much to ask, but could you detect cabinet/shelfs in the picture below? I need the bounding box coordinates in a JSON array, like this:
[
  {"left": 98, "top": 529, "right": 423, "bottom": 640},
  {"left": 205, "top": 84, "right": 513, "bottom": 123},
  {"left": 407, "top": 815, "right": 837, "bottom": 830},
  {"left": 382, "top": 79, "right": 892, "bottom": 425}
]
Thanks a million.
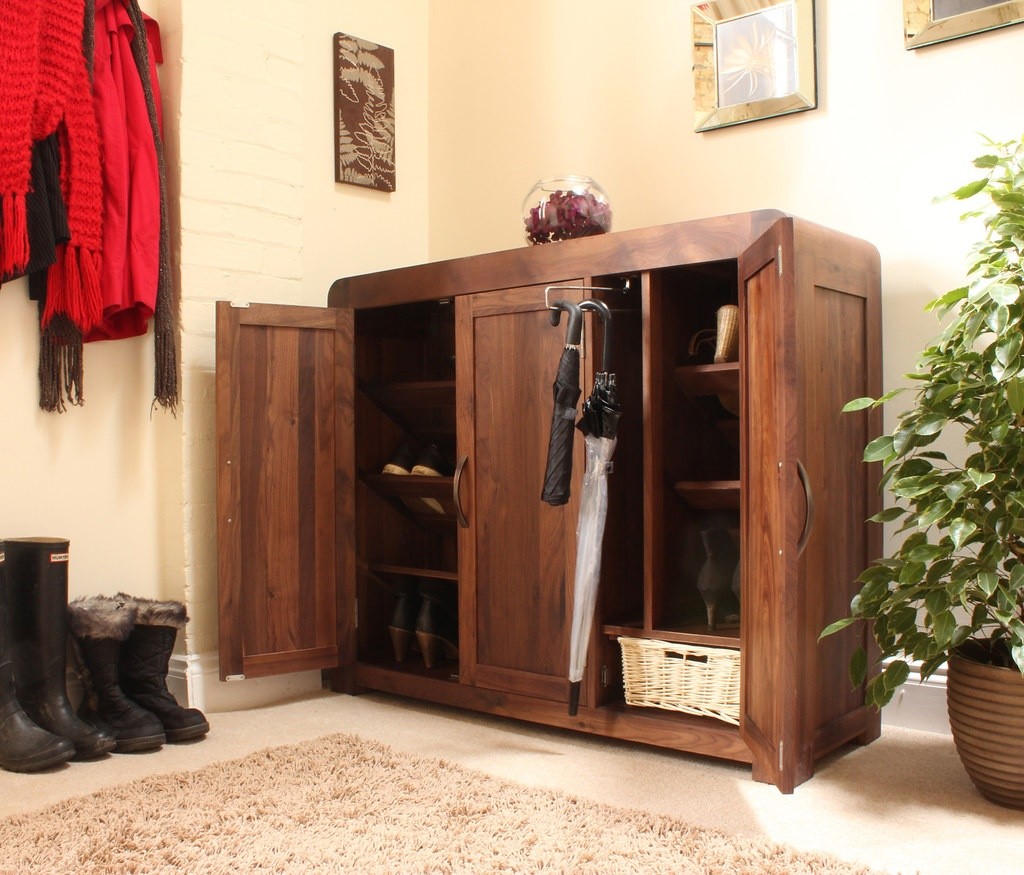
[{"left": 214, "top": 207, "right": 885, "bottom": 795}]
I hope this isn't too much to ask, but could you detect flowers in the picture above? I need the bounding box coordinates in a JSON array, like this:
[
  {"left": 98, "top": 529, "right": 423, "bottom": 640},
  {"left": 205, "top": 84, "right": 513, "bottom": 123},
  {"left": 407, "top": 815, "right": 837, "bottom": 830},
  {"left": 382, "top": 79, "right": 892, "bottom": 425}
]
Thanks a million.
[{"left": 523, "top": 191, "right": 613, "bottom": 246}]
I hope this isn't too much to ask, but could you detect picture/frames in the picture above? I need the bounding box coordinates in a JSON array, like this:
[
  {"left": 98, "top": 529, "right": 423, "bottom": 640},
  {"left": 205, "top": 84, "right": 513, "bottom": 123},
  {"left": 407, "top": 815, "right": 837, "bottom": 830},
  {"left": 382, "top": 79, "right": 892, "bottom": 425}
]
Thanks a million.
[
  {"left": 903, "top": 0, "right": 1024, "bottom": 51},
  {"left": 691, "top": 0, "right": 819, "bottom": 132}
]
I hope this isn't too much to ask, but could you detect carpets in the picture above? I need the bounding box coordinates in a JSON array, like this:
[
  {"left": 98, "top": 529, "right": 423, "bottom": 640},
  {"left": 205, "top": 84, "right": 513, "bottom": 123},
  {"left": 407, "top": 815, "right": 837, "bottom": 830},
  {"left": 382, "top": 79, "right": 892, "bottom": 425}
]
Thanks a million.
[{"left": 0, "top": 732, "right": 952, "bottom": 875}]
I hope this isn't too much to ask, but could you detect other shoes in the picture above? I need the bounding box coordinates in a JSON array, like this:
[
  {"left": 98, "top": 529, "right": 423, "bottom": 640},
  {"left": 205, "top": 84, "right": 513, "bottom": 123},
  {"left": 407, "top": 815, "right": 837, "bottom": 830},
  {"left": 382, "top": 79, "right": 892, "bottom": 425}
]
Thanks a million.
[{"left": 381, "top": 439, "right": 456, "bottom": 514}]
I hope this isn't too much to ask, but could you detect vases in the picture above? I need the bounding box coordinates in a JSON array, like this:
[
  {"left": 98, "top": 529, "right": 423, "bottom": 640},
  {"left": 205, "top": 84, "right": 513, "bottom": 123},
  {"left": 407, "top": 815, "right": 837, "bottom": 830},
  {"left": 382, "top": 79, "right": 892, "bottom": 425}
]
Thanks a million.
[{"left": 520, "top": 177, "right": 616, "bottom": 247}]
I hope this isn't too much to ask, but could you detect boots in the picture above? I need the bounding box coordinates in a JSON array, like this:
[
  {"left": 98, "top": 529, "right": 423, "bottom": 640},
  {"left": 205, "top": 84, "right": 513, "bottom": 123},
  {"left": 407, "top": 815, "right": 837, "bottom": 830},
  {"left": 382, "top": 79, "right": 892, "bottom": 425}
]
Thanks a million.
[
  {"left": 0, "top": 537, "right": 118, "bottom": 760},
  {"left": 698, "top": 523, "right": 736, "bottom": 631},
  {"left": 64, "top": 593, "right": 166, "bottom": 751},
  {"left": 113, "top": 593, "right": 209, "bottom": 741},
  {"left": 1, "top": 553, "right": 76, "bottom": 771}
]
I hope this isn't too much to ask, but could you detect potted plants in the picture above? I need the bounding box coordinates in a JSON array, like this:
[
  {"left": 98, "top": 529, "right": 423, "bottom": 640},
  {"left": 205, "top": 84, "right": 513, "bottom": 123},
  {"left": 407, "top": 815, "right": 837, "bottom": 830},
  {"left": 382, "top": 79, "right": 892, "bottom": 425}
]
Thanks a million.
[{"left": 814, "top": 131, "right": 1024, "bottom": 813}]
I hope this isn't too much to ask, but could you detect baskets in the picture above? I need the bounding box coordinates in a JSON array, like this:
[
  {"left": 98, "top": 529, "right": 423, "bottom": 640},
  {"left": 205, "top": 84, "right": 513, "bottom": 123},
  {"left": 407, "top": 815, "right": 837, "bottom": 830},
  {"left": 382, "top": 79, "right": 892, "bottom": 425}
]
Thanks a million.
[{"left": 616, "top": 637, "right": 742, "bottom": 728}]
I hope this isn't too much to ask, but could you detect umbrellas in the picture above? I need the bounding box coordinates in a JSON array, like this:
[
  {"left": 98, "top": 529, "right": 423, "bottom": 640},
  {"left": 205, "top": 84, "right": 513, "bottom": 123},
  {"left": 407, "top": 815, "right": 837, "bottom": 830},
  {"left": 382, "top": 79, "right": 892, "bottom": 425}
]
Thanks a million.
[
  {"left": 567, "top": 372, "right": 626, "bottom": 718},
  {"left": 538, "top": 343, "right": 584, "bottom": 508}
]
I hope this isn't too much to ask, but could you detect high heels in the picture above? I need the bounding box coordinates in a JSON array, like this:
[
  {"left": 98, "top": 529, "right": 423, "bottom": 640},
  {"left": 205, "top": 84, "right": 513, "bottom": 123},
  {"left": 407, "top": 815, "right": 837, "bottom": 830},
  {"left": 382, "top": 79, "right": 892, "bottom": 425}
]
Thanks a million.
[{"left": 388, "top": 589, "right": 460, "bottom": 669}]
